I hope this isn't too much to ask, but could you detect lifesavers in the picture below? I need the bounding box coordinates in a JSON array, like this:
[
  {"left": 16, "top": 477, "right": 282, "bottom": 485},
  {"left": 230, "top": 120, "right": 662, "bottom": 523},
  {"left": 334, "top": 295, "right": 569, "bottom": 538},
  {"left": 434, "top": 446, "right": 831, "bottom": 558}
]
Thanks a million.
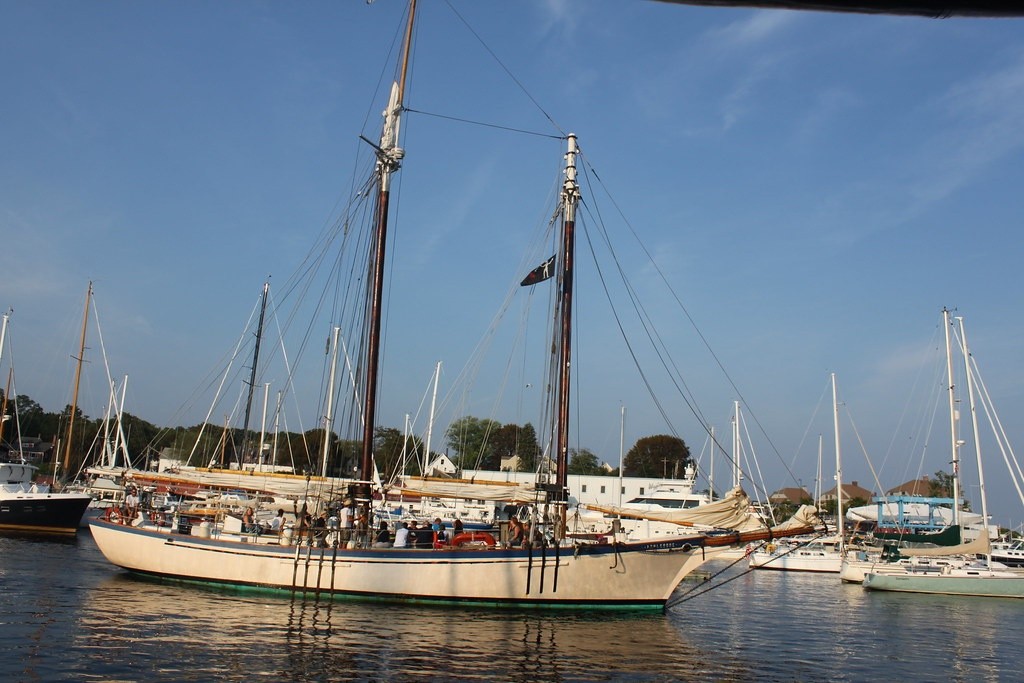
[
  {"left": 766, "top": 546, "right": 775, "bottom": 554},
  {"left": 106, "top": 508, "right": 125, "bottom": 525},
  {"left": 150, "top": 511, "right": 166, "bottom": 525}
]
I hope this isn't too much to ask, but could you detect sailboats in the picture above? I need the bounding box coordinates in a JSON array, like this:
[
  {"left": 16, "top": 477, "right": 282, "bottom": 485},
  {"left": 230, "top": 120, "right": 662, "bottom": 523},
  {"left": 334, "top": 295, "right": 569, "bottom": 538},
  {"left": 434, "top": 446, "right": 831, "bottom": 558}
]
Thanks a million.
[{"left": 0, "top": 0, "right": 1024, "bottom": 619}]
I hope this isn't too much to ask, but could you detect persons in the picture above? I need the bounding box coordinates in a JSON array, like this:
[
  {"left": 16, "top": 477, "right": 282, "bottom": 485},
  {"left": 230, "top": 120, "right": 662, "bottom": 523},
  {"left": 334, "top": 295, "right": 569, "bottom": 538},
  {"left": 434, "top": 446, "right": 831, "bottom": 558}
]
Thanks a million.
[
  {"left": 241, "top": 507, "right": 256, "bottom": 533},
  {"left": 123, "top": 487, "right": 141, "bottom": 526},
  {"left": 271, "top": 508, "right": 286, "bottom": 532},
  {"left": 297, "top": 498, "right": 547, "bottom": 549}
]
